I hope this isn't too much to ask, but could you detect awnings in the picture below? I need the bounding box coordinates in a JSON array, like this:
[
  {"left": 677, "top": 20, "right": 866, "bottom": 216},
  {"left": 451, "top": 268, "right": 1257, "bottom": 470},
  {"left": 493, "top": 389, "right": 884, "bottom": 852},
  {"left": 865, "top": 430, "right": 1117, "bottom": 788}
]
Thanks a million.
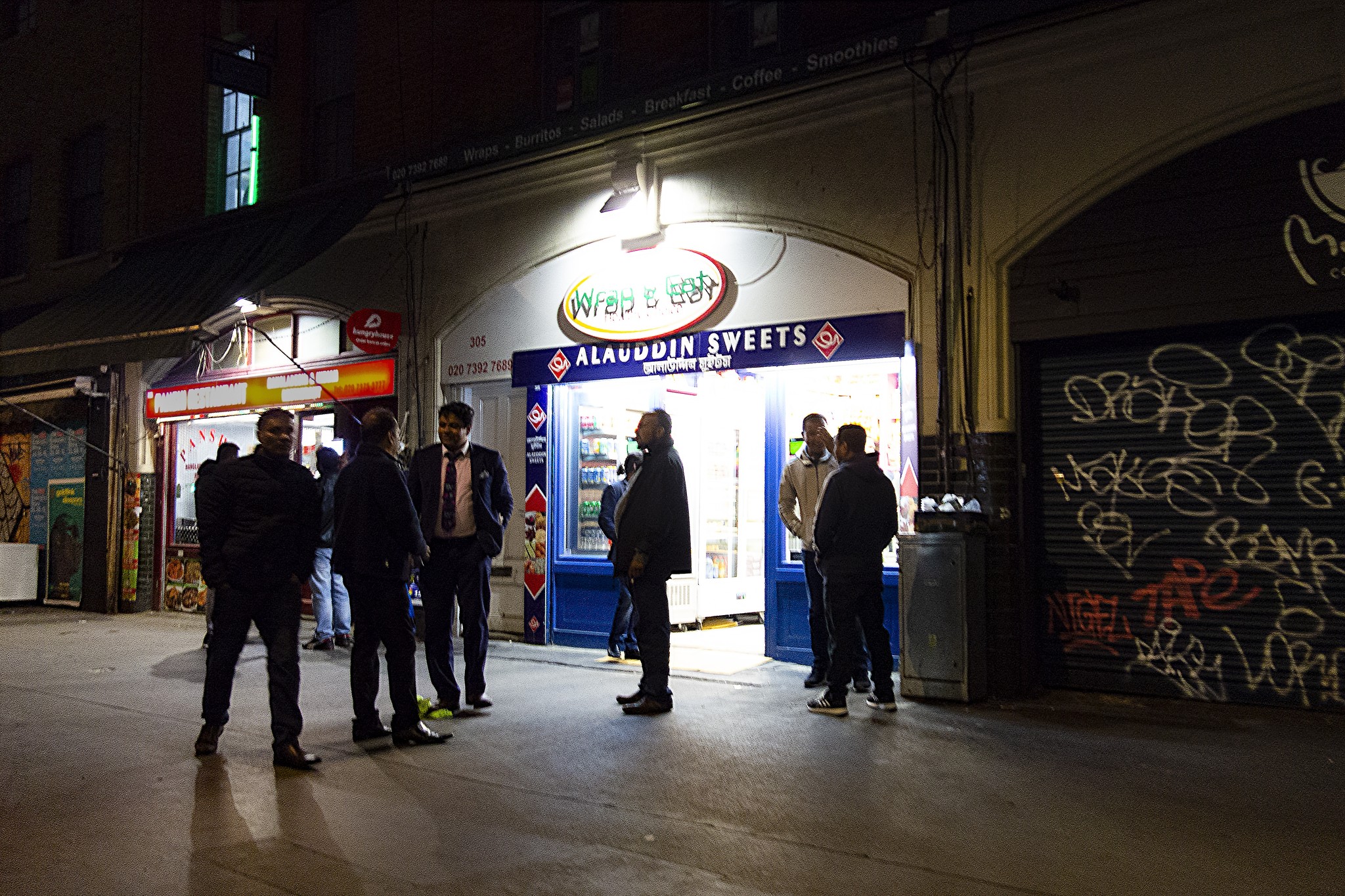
[{"left": 0, "top": 177, "right": 390, "bottom": 374}]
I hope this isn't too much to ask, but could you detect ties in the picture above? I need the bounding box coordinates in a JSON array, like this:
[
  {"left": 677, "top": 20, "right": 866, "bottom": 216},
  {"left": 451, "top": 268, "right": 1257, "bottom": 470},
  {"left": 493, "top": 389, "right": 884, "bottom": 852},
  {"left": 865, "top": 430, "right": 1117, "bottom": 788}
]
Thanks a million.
[{"left": 440, "top": 451, "right": 462, "bottom": 533}]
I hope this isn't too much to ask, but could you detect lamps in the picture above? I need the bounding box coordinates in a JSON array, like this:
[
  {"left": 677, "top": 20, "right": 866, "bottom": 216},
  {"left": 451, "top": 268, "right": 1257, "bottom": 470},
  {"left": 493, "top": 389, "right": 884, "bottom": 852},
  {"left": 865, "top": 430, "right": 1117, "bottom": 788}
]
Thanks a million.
[{"left": 590, "top": 155, "right": 665, "bottom": 249}]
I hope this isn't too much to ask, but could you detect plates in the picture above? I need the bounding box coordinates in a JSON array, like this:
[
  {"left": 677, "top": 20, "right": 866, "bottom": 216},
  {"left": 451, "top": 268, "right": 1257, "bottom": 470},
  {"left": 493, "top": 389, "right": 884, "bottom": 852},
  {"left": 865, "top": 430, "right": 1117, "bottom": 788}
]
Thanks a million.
[
  {"left": 166, "top": 563, "right": 184, "bottom": 581},
  {"left": 181, "top": 587, "right": 198, "bottom": 613},
  {"left": 166, "top": 585, "right": 183, "bottom": 610}
]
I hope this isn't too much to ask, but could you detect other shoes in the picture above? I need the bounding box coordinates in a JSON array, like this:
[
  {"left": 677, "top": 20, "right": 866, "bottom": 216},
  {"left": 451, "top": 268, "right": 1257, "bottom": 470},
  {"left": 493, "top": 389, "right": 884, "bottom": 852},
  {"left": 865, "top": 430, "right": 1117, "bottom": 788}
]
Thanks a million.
[
  {"left": 616, "top": 688, "right": 647, "bottom": 704},
  {"left": 335, "top": 634, "right": 351, "bottom": 646},
  {"left": 203, "top": 633, "right": 213, "bottom": 649},
  {"left": 303, "top": 637, "right": 335, "bottom": 651},
  {"left": 440, "top": 690, "right": 461, "bottom": 710},
  {"left": 473, "top": 692, "right": 493, "bottom": 708},
  {"left": 194, "top": 724, "right": 224, "bottom": 753},
  {"left": 622, "top": 695, "right": 673, "bottom": 715},
  {"left": 271, "top": 738, "right": 322, "bottom": 766}
]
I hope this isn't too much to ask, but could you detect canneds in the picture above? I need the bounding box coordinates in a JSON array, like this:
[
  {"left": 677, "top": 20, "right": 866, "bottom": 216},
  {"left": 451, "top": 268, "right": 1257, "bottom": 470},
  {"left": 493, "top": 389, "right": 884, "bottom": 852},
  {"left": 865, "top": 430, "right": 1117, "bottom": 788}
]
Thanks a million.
[
  {"left": 580, "top": 437, "right": 618, "bottom": 455},
  {"left": 581, "top": 467, "right": 604, "bottom": 484},
  {"left": 602, "top": 465, "right": 617, "bottom": 483},
  {"left": 582, "top": 501, "right": 601, "bottom": 515}
]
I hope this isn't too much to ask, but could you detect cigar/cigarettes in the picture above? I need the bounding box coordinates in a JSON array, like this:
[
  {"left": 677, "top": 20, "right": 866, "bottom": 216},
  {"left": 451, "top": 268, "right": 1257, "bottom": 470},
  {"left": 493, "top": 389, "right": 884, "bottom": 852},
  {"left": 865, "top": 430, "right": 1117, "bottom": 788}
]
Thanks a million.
[{"left": 629, "top": 578, "right": 635, "bottom": 585}]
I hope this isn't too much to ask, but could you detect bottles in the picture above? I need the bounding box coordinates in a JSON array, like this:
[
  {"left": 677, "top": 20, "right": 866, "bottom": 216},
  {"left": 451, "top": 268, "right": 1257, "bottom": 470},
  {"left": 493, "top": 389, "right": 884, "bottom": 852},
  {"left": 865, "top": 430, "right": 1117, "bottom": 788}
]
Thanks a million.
[{"left": 581, "top": 526, "right": 610, "bottom": 550}]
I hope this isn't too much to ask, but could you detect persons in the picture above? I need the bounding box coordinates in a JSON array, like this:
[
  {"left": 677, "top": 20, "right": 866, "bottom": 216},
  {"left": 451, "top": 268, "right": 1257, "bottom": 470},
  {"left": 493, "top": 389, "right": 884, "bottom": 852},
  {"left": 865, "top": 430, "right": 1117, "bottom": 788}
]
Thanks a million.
[
  {"left": 408, "top": 402, "right": 514, "bottom": 714},
  {"left": 300, "top": 447, "right": 352, "bottom": 651},
  {"left": 194, "top": 443, "right": 242, "bottom": 649},
  {"left": 806, "top": 425, "right": 897, "bottom": 718},
  {"left": 779, "top": 413, "right": 873, "bottom": 693},
  {"left": 597, "top": 453, "right": 646, "bottom": 659},
  {"left": 607, "top": 408, "right": 691, "bottom": 714},
  {"left": 329, "top": 407, "right": 455, "bottom": 747},
  {"left": 194, "top": 408, "right": 322, "bottom": 767}
]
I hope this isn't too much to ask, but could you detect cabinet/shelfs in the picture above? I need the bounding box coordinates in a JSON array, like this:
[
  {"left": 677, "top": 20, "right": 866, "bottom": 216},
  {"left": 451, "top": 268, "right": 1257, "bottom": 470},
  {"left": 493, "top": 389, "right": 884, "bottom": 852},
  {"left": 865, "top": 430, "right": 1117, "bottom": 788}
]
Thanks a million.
[{"left": 576, "top": 419, "right": 615, "bottom": 557}]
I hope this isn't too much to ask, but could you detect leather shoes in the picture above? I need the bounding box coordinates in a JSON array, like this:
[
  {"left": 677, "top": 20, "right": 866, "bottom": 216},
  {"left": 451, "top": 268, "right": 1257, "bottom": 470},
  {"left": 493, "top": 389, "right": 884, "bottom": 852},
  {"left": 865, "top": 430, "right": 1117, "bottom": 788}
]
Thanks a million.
[
  {"left": 392, "top": 720, "right": 454, "bottom": 749},
  {"left": 350, "top": 710, "right": 391, "bottom": 742},
  {"left": 607, "top": 644, "right": 621, "bottom": 658},
  {"left": 624, "top": 648, "right": 641, "bottom": 660}
]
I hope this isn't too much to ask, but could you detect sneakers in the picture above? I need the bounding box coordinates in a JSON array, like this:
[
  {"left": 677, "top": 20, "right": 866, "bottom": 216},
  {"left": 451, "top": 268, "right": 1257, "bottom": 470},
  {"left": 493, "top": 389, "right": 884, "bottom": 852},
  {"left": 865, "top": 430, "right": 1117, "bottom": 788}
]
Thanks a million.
[
  {"left": 852, "top": 676, "right": 872, "bottom": 692},
  {"left": 806, "top": 690, "right": 848, "bottom": 716},
  {"left": 867, "top": 690, "right": 898, "bottom": 711},
  {"left": 805, "top": 669, "right": 827, "bottom": 687}
]
